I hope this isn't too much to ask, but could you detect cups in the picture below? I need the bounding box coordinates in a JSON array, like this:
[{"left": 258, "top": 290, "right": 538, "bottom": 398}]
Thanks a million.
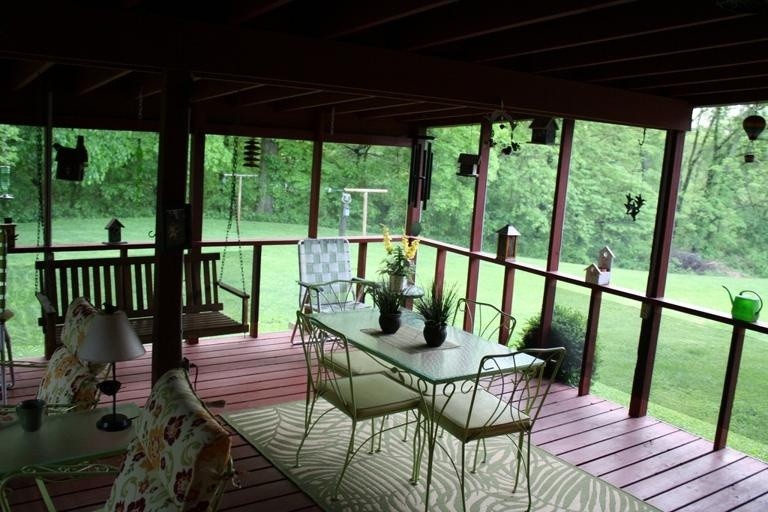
[{"left": 16, "top": 400, "right": 47, "bottom": 432}]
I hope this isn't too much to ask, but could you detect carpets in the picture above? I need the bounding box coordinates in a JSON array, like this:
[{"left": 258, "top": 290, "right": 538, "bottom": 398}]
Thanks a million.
[{"left": 218, "top": 397, "right": 663, "bottom": 512}]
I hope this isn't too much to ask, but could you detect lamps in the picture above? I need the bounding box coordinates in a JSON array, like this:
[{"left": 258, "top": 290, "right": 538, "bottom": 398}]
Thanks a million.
[{"left": 80, "top": 311, "right": 149, "bottom": 431}]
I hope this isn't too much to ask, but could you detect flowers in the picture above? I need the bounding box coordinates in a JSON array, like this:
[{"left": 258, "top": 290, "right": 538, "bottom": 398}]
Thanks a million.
[{"left": 375, "top": 223, "right": 420, "bottom": 277}]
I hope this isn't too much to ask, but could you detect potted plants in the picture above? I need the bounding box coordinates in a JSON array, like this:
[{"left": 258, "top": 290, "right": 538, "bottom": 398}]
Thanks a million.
[
  {"left": 412, "top": 279, "right": 461, "bottom": 347},
  {"left": 365, "top": 276, "right": 414, "bottom": 333}
]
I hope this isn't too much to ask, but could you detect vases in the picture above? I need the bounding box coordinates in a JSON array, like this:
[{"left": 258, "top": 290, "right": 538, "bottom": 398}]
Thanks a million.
[{"left": 389, "top": 274, "right": 405, "bottom": 292}]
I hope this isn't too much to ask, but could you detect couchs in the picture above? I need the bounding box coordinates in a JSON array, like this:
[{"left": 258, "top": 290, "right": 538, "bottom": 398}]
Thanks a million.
[
  {"left": 92, "top": 364, "right": 241, "bottom": 512},
  {"left": 0, "top": 295, "right": 105, "bottom": 426}
]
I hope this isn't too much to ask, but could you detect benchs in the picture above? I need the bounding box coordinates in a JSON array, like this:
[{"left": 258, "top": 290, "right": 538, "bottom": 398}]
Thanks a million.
[{"left": 36, "top": 252, "right": 249, "bottom": 360}]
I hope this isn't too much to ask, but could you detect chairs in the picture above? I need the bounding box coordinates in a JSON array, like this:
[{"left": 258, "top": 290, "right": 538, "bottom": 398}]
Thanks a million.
[
  {"left": 296, "top": 280, "right": 409, "bottom": 451},
  {"left": 377, "top": 297, "right": 518, "bottom": 451},
  {"left": 417, "top": 346, "right": 566, "bottom": 511},
  {"left": 291, "top": 239, "right": 379, "bottom": 346},
  {"left": 295, "top": 311, "right": 423, "bottom": 501}
]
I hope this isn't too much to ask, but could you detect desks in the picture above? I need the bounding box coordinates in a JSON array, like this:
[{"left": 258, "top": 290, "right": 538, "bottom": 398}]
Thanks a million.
[
  {"left": 0, "top": 400, "right": 148, "bottom": 512},
  {"left": 307, "top": 312, "right": 546, "bottom": 484}
]
